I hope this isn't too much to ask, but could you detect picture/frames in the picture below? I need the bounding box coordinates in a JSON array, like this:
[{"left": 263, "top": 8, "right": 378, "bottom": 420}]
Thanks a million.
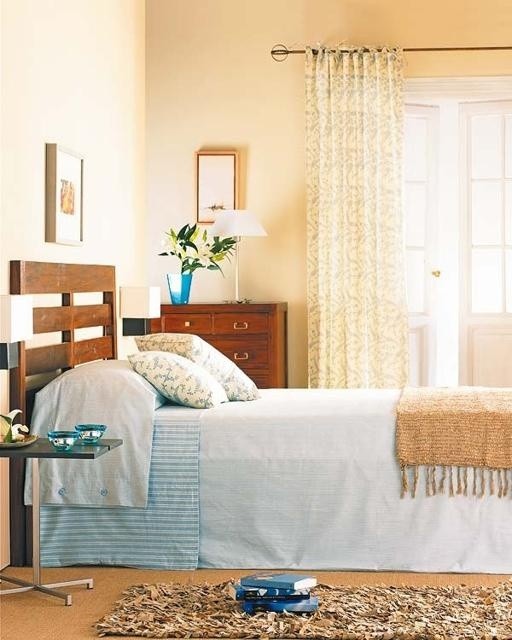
[
  {"left": 194, "top": 151, "right": 239, "bottom": 225},
  {"left": 45, "top": 143, "right": 86, "bottom": 246}
]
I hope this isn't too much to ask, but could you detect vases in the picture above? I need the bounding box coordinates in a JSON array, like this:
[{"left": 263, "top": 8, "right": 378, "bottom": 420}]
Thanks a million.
[{"left": 165, "top": 274, "right": 193, "bottom": 305}]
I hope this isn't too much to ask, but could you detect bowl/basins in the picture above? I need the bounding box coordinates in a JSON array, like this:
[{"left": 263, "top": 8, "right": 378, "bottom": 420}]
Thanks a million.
[
  {"left": 46, "top": 430, "right": 78, "bottom": 451},
  {"left": 74, "top": 423, "right": 108, "bottom": 444}
]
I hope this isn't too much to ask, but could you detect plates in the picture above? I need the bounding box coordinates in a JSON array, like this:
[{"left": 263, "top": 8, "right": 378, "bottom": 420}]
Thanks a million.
[{"left": 0, "top": 435, "right": 40, "bottom": 448}]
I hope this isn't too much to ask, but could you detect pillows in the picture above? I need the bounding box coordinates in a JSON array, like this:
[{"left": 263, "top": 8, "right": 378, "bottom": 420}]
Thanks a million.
[
  {"left": 37, "top": 355, "right": 167, "bottom": 410},
  {"left": 127, "top": 350, "right": 229, "bottom": 410},
  {"left": 134, "top": 333, "right": 262, "bottom": 402}
]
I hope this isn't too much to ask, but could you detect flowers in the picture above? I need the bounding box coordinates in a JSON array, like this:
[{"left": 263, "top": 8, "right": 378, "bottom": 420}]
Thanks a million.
[{"left": 157, "top": 224, "right": 238, "bottom": 274}]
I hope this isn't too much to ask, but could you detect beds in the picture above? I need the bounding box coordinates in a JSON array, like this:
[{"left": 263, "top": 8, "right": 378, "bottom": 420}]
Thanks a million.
[{"left": 9, "top": 259, "right": 512, "bottom": 575}]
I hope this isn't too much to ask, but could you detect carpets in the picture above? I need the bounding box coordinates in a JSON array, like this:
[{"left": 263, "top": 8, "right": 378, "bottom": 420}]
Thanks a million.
[{"left": 95, "top": 580, "right": 511, "bottom": 640}]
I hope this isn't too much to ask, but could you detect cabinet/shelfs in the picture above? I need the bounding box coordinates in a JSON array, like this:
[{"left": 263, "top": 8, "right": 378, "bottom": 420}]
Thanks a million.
[{"left": 159, "top": 303, "right": 290, "bottom": 388}]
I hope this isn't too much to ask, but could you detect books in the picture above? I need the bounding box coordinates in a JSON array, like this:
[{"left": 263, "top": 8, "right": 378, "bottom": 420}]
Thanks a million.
[
  {"left": 239, "top": 603, "right": 317, "bottom": 615},
  {"left": 239, "top": 569, "right": 321, "bottom": 593},
  {"left": 226, "top": 584, "right": 314, "bottom": 601}
]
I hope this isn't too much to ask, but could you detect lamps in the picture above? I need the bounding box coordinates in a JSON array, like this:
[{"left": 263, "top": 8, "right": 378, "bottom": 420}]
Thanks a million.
[
  {"left": 117, "top": 287, "right": 161, "bottom": 334},
  {"left": 208, "top": 210, "right": 268, "bottom": 303},
  {"left": 0, "top": 293, "right": 33, "bottom": 370}
]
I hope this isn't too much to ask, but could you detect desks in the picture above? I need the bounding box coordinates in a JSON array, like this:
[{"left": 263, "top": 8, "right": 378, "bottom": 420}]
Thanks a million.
[{"left": 1, "top": 434, "right": 123, "bottom": 610}]
